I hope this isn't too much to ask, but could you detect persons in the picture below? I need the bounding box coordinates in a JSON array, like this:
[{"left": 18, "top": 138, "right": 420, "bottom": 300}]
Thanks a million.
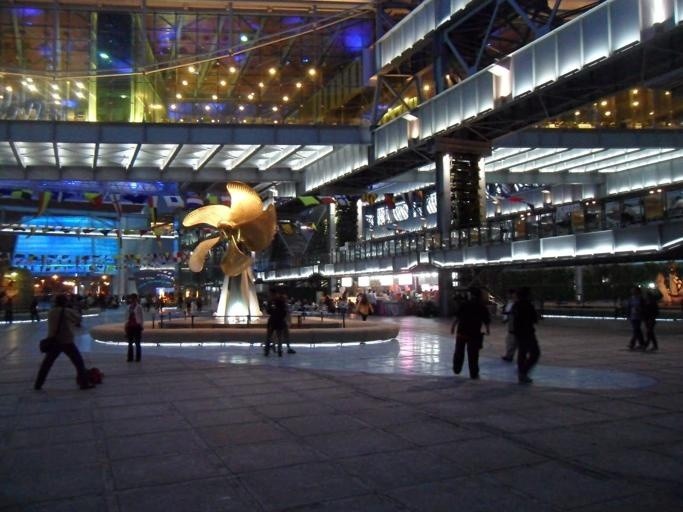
[
  {"left": 67, "top": 293, "right": 118, "bottom": 326},
  {"left": 278, "top": 295, "right": 296, "bottom": 353},
  {"left": 35, "top": 294, "right": 85, "bottom": 388},
  {"left": 626, "top": 287, "right": 644, "bottom": 349},
  {"left": 508, "top": 286, "right": 541, "bottom": 385},
  {"left": 264, "top": 287, "right": 286, "bottom": 356},
  {"left": 2, "top": 297, "right": 14, "bottom": 325},
  {"left": 502, "top": 287, "right": 517, "bottom": 361},
  {"left": 291, "top": 290, "right": 439, "bottom": 318},
  {"left": 140, "top": 292, "right": 203, "bottom": 311},
  {"left": 31, "top": 296, "right": 39, "bottom": 322},
  {"left": 451, "top": 288, "right": 490, "bottom": 379},
  {"left": 122, "top": 293, "right": 144, "bottom": 363},
  {"left": 639, "top": 292, "right": 659, "bottom": 351}
]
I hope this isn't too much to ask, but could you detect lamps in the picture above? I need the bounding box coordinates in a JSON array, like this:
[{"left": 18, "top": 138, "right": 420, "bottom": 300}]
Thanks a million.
[
  {"left": 487, "top": 57, "right": 511, "bottom": 78},
  {"left": 401, "top": 107, "right": 419, "bottom": 122}
]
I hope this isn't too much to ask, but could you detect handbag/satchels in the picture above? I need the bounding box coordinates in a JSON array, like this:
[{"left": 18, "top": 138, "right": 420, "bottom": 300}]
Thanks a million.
[{"left": 40, "top": 338, "right": 55, "bottom": 352}]
